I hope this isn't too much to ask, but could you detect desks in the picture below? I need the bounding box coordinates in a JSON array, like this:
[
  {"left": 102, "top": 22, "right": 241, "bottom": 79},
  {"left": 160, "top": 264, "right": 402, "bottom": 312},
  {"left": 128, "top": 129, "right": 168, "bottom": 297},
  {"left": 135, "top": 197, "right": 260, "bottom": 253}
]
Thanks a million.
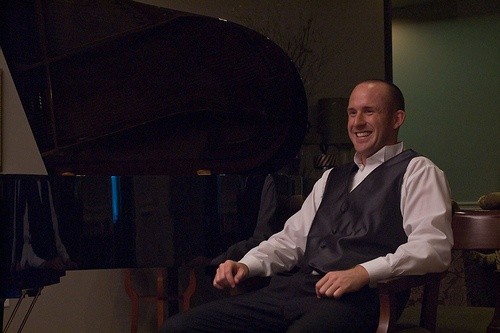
[{"left": 121, "top": 264, "right": 197, "bottom": 333}]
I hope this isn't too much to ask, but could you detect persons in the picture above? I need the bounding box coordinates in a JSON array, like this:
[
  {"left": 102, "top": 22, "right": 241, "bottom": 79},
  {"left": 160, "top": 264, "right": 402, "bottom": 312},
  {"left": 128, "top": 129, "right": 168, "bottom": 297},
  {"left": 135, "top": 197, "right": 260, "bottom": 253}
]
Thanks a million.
[
  {"left": 159, "top": 79, "right": 454, "bottom": 333},
  {"left": 20, "top": 137, "right": 280, "bottom": 270}
]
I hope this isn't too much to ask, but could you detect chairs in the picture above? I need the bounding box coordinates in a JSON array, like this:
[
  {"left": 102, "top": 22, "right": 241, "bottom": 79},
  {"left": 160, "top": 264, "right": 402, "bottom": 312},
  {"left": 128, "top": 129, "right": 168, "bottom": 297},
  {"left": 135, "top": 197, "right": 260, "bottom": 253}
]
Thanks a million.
[{"left": 376, "top": 200, "right": 500, "bottom": 333}]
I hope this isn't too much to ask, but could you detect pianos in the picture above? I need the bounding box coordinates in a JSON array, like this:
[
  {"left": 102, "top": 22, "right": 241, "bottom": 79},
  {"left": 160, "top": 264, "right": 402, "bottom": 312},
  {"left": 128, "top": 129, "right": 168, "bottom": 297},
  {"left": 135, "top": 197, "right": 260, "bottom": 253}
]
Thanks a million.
[{"left": 0, "top": 0, "right": 310, "bottom": 333}]
